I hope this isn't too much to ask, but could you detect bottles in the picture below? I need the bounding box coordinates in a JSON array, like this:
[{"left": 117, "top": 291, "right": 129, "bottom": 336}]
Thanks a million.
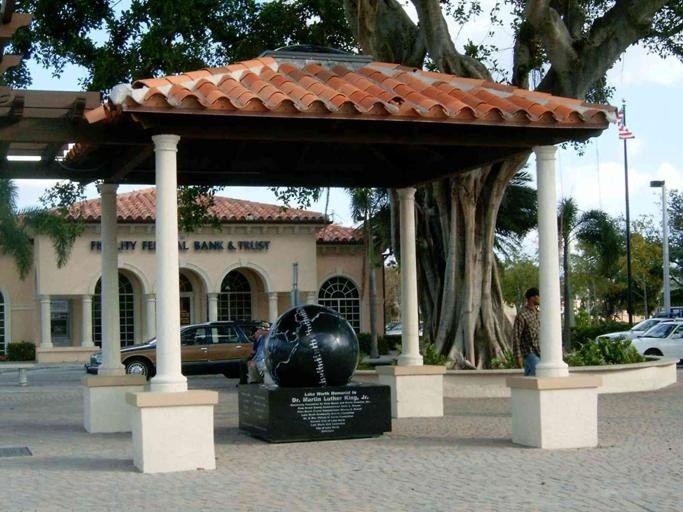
[
  {"left": 526, "top": 287, "right": 540, "bottom": 297},
  {"left": 257, "top": 320, "right": 271, "bottom": 332}
]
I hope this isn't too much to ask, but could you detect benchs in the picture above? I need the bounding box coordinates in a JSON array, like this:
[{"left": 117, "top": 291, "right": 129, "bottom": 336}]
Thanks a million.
[{"left": 615, "top": 106, "right": 635, "bottom": 139}]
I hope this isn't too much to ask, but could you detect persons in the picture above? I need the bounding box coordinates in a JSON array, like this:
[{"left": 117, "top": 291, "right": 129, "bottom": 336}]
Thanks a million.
[
  {"left": 236, "top": 322, "right": 270, "bottom": 388},
  {"left": 512, "top": 288, "right": 540, "bottom": 376}
]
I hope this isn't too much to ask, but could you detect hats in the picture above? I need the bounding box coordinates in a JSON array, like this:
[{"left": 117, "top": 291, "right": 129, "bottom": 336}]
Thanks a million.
[
  {"left": 386, "top": 319, "right": 428, "bottom": 339},
  {"left": 593, "top": 305, "right": 682, "bottom": 367}
]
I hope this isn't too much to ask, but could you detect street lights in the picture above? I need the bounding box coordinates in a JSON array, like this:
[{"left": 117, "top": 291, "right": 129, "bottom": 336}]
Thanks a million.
[{"left": 240, "top": 357, "right": 263, "bottom": 384}]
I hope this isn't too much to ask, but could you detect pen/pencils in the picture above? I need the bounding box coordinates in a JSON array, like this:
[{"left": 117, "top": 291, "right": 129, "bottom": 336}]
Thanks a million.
[{"left": 83, "top": 319, "right": 270, "bottom": 381}]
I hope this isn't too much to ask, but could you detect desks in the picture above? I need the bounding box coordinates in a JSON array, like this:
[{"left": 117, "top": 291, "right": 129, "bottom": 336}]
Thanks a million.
[{"left": 649, "top": 179, "right": 669, "bottom": 315}]
